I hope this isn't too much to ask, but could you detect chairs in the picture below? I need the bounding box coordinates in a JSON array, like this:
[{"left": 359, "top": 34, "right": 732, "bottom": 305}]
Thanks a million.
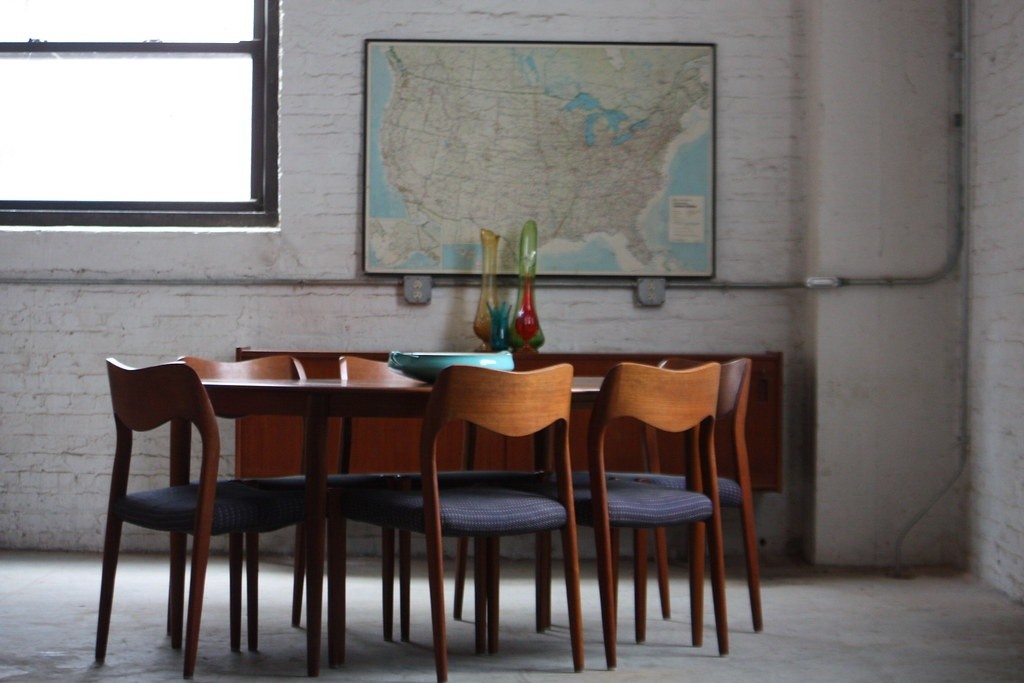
[{"left": 81, "top": 349, "right": 764, "bottom": 682}]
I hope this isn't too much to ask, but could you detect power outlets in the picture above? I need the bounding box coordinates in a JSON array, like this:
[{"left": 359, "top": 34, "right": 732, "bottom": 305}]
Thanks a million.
[
  {"left": 400, "top": 275, "right": 436, "bottom": 305},
  {"left": 635, "top": 277, "right": 669, "bottom": 306}
]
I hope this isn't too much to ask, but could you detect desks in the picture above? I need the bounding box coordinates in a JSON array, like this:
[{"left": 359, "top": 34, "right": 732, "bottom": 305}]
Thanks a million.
[{"left": 172, "top": 372, "right": 707, "bottom": 670}]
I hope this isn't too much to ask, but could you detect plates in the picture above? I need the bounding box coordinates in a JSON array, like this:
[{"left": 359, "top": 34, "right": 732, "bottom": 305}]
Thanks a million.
[{"left": 387, "top": 350, "right": 515, "bottom": 385}]
